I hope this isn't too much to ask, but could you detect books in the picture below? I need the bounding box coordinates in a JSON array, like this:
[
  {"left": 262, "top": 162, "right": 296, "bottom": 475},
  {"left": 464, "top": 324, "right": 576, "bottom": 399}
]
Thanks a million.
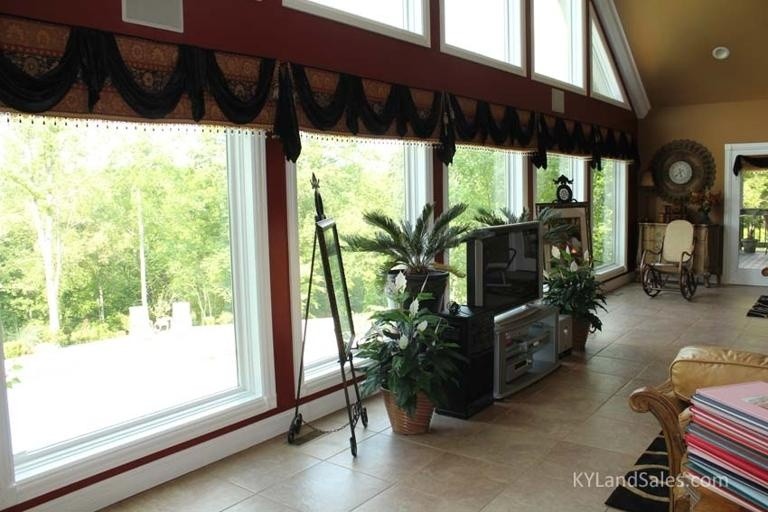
[{"left": 684, "top": 380, "right": 768, "bottom": 512}]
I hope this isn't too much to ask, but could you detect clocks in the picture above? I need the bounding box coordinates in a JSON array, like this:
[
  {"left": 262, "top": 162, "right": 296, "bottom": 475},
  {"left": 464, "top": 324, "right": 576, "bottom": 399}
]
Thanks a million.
[{"left": 667, "top": 159, "right": 693, "bottom": 186}]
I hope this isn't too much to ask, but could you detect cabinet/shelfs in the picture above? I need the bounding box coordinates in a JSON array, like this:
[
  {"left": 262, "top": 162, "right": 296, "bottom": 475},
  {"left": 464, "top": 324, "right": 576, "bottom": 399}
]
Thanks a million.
[
  {"left": 634, "top": 223, "right": 724, "bottom": 288},
  {"left": 492, "top": 304, "right": 563, "bottom": 399}
]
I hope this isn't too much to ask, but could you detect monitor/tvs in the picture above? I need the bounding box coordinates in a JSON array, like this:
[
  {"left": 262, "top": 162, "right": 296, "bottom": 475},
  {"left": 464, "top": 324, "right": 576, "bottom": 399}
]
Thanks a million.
[{"left": 467, "top": 221, "right": 544, "bottom": 314}]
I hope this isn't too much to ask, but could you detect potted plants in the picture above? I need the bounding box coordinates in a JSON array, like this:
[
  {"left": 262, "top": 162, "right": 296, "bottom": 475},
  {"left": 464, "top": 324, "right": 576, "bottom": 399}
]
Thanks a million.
[
  {"left": 739, "top": 214, "right": 763, "bottom": 253},
  {"left": 337, "top": 202, "right": 472, "bottom": 314}
]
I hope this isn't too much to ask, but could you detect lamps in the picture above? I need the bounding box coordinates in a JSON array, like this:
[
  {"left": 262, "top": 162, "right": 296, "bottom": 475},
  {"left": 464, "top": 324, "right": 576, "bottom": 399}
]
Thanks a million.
[{"left": 637, "top": 170, "right": 656, "bottom": 223}]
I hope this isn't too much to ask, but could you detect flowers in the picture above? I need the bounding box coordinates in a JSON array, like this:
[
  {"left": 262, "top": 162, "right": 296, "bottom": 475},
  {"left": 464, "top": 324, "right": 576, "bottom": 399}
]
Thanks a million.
[
  {"left": 348, "top": 287, "right": 471, "bottom": 422},
  {"left": 543, "top": 243, "right": 609, "bottom": 334},
  {"left": 688, "top": 189, "right": 722, "bottom": 212}
]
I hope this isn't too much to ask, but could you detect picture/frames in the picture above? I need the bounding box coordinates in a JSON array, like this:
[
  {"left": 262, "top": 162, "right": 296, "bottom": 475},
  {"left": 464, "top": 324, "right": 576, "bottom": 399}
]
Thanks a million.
[
  {"left": 534, "top": 200, "right": 594, "bottom": 276},
  {"left": 316, "top": 216, "right": 356, "bottom": 365}
]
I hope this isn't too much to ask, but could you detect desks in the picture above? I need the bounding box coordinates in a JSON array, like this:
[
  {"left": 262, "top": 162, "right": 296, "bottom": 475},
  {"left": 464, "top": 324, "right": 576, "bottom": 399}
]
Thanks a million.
[{"left": 678, "top": 450, "right": 748, "bottom": 512}]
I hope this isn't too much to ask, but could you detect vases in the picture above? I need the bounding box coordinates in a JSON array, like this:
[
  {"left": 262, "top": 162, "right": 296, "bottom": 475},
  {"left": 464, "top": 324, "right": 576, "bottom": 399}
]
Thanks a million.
[
  {"left": 697, "top": 207, "right": 709, "bottom": 224},
  {"left": 559, "top": 310, "right": 590, "bottom": 351},
  {"left": 379, "top": 383, "right": 436, "bottom": 436}
]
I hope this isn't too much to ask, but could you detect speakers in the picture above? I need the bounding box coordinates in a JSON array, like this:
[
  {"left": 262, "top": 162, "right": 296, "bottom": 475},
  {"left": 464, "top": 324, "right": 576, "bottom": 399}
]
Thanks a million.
[{"left": 558, "top": 313, "right": 573, "bottom": 359}]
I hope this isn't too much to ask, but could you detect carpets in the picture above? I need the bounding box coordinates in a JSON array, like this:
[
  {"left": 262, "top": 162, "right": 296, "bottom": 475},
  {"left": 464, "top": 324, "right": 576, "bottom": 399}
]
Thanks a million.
[
  {"left": 746, "top": 294, "right": 768, "bottom": 318},
  {"left": 604, "top": 430, "right": 671, "bottom": 512}
]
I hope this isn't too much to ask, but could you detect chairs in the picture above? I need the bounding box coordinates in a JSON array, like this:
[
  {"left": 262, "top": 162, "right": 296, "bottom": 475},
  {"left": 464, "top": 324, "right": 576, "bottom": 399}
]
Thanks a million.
[
  {"left": 638, "top": 219, "right": 698, "bottom": 301},
  {"left": 628, "top": 345, "right": 768, "bottom": 512}
]
